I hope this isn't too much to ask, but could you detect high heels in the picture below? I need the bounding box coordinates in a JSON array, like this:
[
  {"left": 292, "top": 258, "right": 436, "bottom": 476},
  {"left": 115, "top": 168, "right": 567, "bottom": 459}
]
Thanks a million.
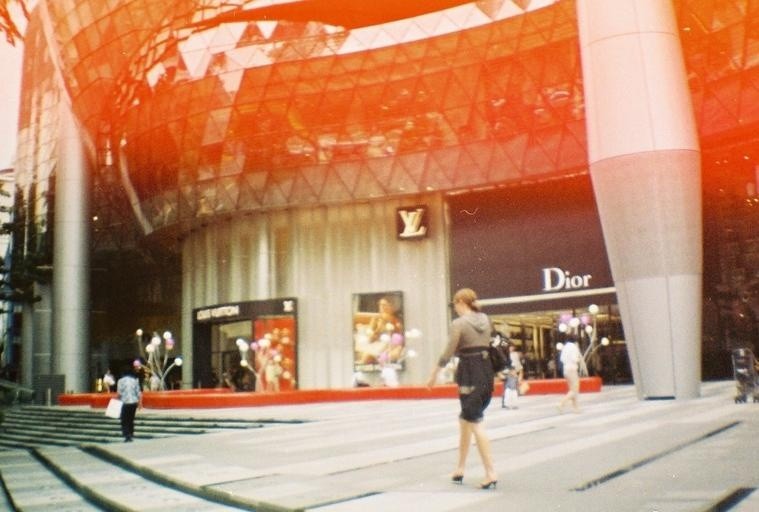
[
  {"left": 451, "top": 474, "right": 464, "bottom": 485},
  {"left": 480, "top": 478, "right": 497, "bottom": 489}
]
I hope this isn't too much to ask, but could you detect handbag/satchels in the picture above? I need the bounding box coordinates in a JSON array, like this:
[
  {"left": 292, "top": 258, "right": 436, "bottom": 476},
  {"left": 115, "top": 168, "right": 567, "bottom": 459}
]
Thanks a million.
[
  {"left": 105, "top": 399, "right": 123, "bottom": 418},
  {"left": 489, "top": 345, "right": 512, "bottom": 373}
]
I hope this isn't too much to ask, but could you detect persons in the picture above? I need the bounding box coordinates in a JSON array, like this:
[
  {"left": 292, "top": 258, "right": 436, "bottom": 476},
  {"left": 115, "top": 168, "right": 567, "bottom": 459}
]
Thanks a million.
[
  {"left": 557, "top": 335, "right": 589, "bottom": 415},
  {"left": 357, "top": 297, "right": 403, "bottom": 364},
  {"left": 116, "top": 366, "right": 144, "bottom": 442},
  {"left": 499, "top": 334, "right": 522, "bottom": 409},
  {"left": 427, "top": 288, "right": 497, "bottom": 489},
  {"left": 103, "top": 369, "right": 115, "bottom": 393},
  {"left": 149, "top": 375, "right": 160, "bottom": 391}
]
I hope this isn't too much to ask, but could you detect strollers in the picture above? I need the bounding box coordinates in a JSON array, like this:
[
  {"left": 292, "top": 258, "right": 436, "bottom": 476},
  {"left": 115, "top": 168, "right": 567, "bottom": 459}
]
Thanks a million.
[{"left": 731, "top": 346, "right": 759, "bottom": 404}]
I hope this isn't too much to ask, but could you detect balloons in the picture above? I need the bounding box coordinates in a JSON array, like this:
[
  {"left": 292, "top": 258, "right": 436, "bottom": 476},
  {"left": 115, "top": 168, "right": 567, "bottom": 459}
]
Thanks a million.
[
  {"left": 368, "top": 321, "right": 419, "bottom": 360},
  {"left": 236, "top": 328, "right": 293, "bottom": 380},
  {"left": 558, "top": 303, "right": 610, "bottom": 346},
  {"left": 132, "top": 327, "right": 184, "bottom": 368}
]
